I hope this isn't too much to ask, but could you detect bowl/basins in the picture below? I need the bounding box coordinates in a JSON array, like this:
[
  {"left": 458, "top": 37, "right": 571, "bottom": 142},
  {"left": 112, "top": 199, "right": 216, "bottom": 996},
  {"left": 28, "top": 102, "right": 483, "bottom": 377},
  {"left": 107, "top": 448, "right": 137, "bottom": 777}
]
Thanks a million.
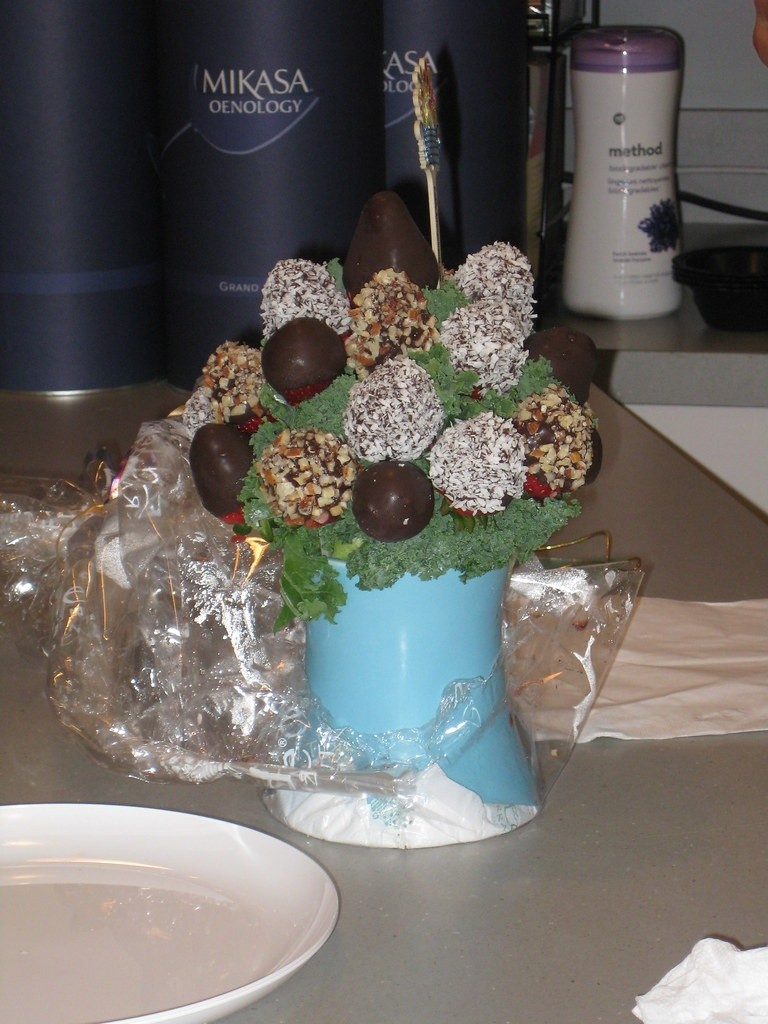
[{"left": 671, "top": 244, "right": 768, "bottom": 332}]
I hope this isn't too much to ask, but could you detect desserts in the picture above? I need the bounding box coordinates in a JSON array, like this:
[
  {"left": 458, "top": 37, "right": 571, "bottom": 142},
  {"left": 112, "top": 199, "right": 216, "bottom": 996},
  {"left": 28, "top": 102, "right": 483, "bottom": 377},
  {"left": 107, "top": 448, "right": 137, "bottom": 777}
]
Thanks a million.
[{"left": 185, "top": 188, "right": 603, "bottom": 582}]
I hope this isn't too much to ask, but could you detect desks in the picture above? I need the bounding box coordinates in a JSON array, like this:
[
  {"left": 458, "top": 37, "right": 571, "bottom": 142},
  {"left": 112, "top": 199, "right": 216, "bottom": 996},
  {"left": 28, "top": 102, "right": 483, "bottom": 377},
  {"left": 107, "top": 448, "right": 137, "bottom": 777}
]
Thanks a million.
[{"left": 0, "top": 378, "right": 768, "bottom": 1024}]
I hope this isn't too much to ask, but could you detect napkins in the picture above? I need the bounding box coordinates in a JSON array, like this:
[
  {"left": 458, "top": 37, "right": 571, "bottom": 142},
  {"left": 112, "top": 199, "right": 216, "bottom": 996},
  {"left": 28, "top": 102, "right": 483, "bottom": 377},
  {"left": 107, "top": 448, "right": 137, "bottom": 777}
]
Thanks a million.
[{"left": 501, "top": 593, "right": 768, "bottom": 741}]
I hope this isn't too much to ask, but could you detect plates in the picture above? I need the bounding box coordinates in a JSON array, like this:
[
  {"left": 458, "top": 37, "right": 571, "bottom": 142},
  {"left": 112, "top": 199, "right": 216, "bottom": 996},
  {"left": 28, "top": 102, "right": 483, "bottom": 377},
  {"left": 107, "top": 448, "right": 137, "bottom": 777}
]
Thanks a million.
[{"left": 0, "top": 803, "right": 344, "bottom": 1024}]
